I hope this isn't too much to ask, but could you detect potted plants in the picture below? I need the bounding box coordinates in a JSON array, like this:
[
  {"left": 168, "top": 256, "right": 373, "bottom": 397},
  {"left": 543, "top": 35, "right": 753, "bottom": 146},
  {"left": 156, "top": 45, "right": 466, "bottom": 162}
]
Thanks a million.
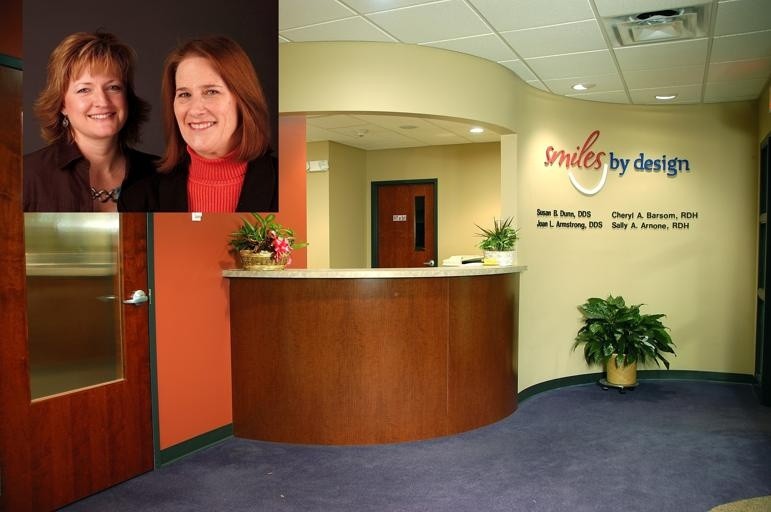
[
  {"left": 570, "top": 294, "right": 681, "bottom": 386},
  {"left": 472, "top": 215, "right": 524, "bottom": 265}
]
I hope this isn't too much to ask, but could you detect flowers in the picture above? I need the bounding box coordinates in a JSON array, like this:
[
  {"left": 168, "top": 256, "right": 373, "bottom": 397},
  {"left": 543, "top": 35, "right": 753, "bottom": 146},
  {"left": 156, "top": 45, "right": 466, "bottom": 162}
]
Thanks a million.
[{"left": 226, "top": 211, "right": 309, "bottom": 271}]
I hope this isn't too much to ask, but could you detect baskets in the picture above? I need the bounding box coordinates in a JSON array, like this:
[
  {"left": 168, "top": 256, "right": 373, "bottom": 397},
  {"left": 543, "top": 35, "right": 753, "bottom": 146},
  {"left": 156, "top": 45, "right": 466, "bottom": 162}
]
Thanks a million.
[{"left": 237, "top": 249, "right": 292, "bottom": 272}]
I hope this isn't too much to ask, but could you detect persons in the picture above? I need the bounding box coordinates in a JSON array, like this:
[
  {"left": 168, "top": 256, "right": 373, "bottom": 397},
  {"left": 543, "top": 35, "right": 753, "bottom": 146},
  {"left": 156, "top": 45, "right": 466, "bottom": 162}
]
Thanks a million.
[
  {"left": 22, "top": 27, "right": 163, "bottom": 213},
  {"left": 155, "top": 35, "right": 277, "bottom": 212}
]
